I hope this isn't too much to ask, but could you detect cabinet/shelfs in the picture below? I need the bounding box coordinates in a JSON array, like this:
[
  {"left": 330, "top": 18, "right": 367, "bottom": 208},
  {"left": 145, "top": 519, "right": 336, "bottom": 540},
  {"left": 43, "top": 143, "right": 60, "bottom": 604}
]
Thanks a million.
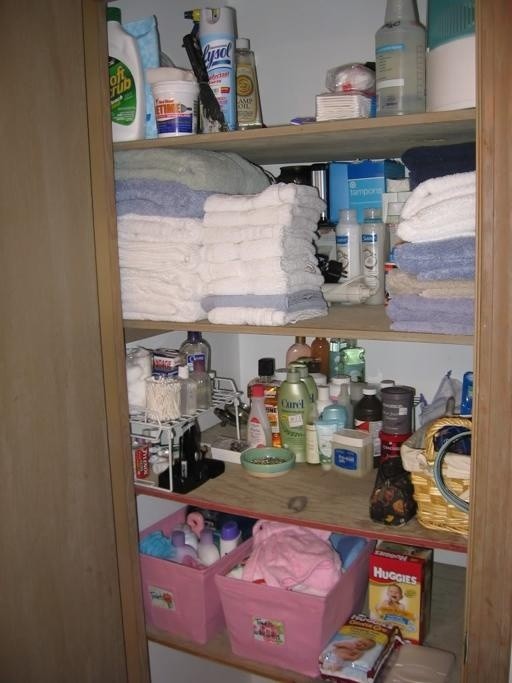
[
  {"left": 102, "top": 1, "right": 481, "bottom": 681},
  {"left": 2, "top": 0, "right": 152, "bottom": 683}
]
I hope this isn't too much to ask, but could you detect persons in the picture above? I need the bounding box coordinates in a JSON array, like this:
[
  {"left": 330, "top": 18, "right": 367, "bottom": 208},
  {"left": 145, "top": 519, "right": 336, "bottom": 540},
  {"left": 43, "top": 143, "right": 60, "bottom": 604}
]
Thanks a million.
[
  {"left": 379, "top": 582, "right": 406, "bottom": 613},
  {"left": 323, "top": 638, "right": 376, "bottom": 671}
]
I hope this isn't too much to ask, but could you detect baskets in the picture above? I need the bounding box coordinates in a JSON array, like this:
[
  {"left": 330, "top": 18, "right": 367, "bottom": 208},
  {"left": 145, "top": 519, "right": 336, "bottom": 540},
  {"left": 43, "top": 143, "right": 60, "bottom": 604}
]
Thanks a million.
[{"left": 401, "top": 418, "right": 472, "bottom": 535}]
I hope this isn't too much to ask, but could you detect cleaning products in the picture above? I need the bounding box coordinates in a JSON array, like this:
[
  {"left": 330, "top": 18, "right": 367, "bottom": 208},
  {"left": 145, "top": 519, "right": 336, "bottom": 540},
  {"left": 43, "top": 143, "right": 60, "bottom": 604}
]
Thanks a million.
[{"left": 106, "top": 6, "right": 147, "bottom": 144}]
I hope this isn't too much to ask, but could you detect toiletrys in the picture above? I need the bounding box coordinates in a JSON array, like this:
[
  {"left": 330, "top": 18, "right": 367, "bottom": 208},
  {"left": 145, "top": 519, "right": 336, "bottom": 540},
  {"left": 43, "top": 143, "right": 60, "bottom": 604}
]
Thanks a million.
[
  {"left": 246, "top": 385, "right": 273, "bottom": 450},
  {"left": 197, "top": 529, "right": 219, "bottom": 566},
  {"left": 182, "top": 523, "right": 198, "bottom": 552},
  {"left": 220, "top": 521, "right": 241, "bottom": 558},
  {"left": 296, "top": 357, "right": 318, "bottom": 425},
  {"left": 171, "top": 531, "right": 198, "bottom": 563},
  {"left": 285, "top": 336, "right": 312, "bottom": 369},
  {"left": 277, "top": 361, "right": 312, "bottom": 463}
]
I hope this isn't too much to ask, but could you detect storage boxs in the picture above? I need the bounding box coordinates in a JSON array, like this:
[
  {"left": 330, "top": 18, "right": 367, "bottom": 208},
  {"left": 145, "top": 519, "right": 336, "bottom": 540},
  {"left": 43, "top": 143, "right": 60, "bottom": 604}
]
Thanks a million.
[
  {"left": 216, "top": 520, "right": 377, "bottom": 678},
  {"left": 126, "top": 501, "right": 289, "bottom": 642}
]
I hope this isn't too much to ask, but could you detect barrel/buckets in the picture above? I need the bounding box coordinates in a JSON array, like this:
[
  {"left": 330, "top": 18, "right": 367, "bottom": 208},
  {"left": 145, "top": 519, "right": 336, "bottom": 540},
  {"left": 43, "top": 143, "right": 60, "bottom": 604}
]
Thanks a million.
[{"left": 150, "top": 80, "right": 201, "bottom": 139}]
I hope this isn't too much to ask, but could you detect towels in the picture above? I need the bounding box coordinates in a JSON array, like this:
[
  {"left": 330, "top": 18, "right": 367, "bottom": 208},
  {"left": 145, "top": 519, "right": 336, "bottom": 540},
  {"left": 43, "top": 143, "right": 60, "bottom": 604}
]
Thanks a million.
[
  {"left": 383, "top": 142, "right": 478, "bottom": 332},
  {"left": 113, "top": 145, "right": 330, "bottom": 324}
]
